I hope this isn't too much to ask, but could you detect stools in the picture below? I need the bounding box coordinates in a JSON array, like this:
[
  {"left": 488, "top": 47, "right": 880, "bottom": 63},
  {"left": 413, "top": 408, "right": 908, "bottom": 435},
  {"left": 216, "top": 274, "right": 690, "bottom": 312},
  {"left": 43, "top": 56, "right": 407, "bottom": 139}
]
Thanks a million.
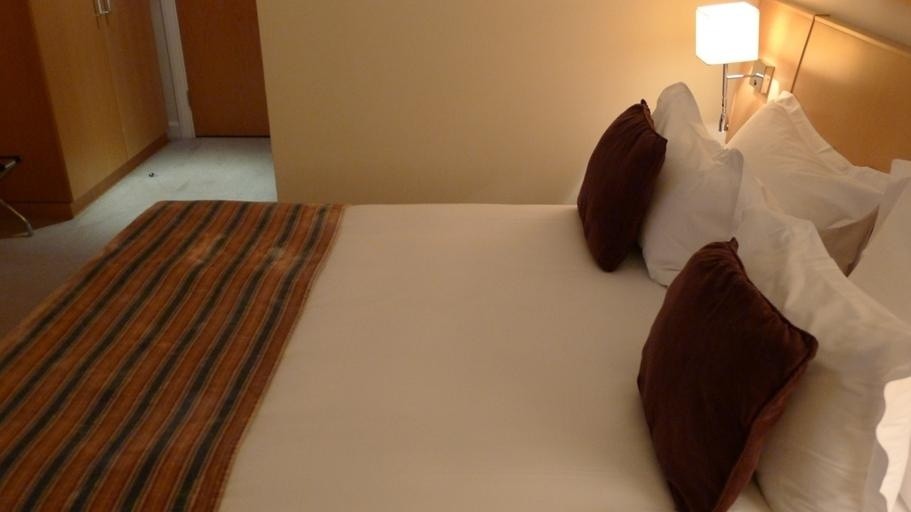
[{"left": 1, "top": 153, "right": 39, "bottom": 239}]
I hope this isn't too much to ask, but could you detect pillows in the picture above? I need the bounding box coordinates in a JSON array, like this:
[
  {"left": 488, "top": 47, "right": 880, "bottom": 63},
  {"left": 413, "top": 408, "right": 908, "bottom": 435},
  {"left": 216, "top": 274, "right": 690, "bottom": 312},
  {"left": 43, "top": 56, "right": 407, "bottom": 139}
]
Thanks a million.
[
  {"left": 635, "top": 233, "right": 823, "bottom": 512},
  {"left": 725, "top": 90, "right": 890, "bottom": 275},
  {"left": 640, "top": 80, "right": 748, "bottom": 288},
  {"left": 845, "top": 156, "right": 908, "bottom": 327},
  {"left": 576, "top": 97, "right": 668, "bottom": 273},
  {"left": 733, "top": 176, "right": 910, "bottom": 512}
]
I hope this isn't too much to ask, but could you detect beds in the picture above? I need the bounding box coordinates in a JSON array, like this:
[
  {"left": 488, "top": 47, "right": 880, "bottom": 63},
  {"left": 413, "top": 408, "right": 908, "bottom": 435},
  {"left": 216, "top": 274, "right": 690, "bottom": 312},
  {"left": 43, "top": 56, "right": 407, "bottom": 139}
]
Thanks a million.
[{"left": 2, "top": 1, "right": 910, "bottom": 512}]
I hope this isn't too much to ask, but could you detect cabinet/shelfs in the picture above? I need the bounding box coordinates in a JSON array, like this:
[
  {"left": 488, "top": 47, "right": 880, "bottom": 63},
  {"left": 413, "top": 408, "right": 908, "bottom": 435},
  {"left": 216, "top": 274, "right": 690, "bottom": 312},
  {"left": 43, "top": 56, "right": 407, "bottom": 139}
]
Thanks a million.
[{"left": 2, "top": 1, "right": 171, "bottom": 224}]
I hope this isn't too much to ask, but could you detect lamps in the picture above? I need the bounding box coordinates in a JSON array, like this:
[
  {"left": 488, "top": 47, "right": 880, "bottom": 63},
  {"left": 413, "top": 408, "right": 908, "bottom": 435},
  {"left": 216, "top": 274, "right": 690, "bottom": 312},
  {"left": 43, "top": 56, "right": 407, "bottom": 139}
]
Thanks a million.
[{"left": 693, "top": 1, "right": 779, "bottom": 134}]
